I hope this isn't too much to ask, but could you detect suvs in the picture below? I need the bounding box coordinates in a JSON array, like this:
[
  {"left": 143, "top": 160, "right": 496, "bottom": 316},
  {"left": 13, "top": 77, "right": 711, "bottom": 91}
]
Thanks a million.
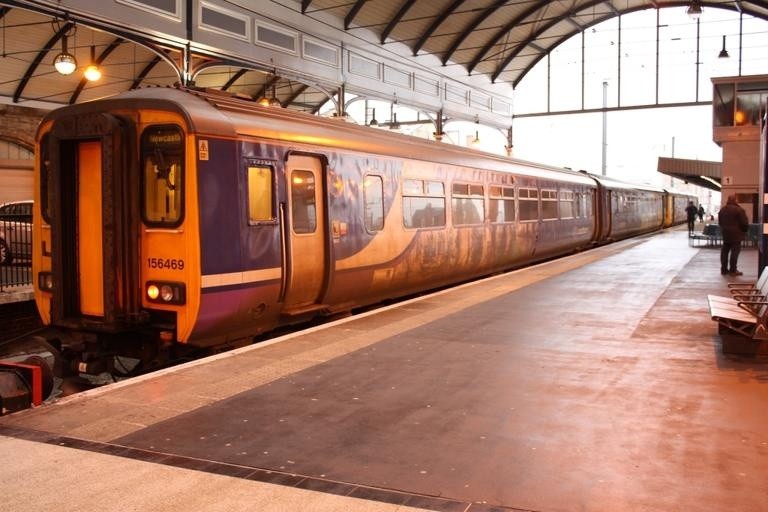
[{"left": 0, "top": 200, "right": 33, "bottom": 266}]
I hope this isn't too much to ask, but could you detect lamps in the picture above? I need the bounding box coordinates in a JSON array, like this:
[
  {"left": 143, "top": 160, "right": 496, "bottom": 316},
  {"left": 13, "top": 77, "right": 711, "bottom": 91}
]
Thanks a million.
[
  {"left": 49, "top": 12, "right": 78, "bottom": 77},
  {"left": 84, "top": 42, "right": 102, "bottom": 83},
  {"left": 684, "top": 0, "right": 703, "bottom": 21},
  {"left": 388, "top": 112, "right": 401, "bottom": 130},
  {"left": 370, "top": 108, "right": 379, "bottom": 129},
  {"left": 718, "top": 35, "right": 730, "bottom": 64},
  {"left": 256, "top": 71, "right": 270, "bottom": 107}
]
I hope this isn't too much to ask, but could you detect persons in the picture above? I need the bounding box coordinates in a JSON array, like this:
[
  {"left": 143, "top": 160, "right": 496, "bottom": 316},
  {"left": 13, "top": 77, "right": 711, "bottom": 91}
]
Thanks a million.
[
  {"left": 685, "top": 200, "right": 697, "bottom": 234},
  {"left": 718, "top": 193, "right": 750, "bottom": 277},
  {"left": 697, "top": 203, "right": 705, "bottom": 221}
]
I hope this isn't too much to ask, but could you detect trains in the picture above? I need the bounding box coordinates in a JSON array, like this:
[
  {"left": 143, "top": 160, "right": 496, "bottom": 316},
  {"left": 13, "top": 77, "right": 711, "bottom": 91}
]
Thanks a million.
[{"left": 30, "top": 85, "right": 700, "bottom": 379}]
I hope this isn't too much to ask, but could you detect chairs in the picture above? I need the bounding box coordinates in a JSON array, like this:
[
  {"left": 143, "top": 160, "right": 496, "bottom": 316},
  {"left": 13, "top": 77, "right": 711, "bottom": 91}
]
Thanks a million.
[
  {"left": 709, "top": 263, "right": 767, "bottom": 362},
  {"left": 688, "top": 218, "right": 763, "bottom": 251}
]
